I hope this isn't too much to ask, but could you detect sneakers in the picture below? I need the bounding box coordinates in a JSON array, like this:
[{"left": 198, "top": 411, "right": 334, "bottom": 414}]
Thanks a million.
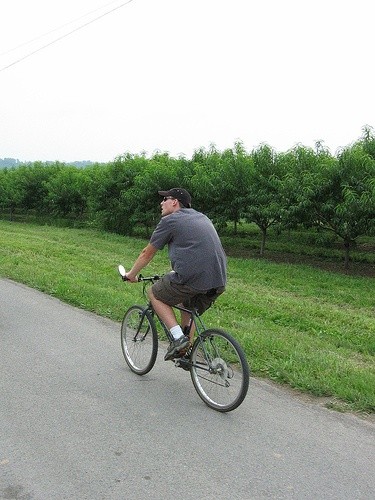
[
  {"left": 175, "top": 357, "right": 191, "bottom": 368},
  {"left": 163, "top": 335, "right": 192, "bottom": 361}
]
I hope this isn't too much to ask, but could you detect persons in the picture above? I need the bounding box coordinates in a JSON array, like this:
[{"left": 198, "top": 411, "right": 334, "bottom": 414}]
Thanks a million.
[{"left": 124, "top": 188, "right": 227, "bottom": 371}]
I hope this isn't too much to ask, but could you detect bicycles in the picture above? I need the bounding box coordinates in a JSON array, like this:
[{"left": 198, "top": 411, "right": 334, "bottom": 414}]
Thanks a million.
[{"left": 119, "top": 265, "right": 250, "bottom": 412}]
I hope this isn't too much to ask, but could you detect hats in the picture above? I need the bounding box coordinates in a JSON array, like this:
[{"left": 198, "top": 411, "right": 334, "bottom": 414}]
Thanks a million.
[{"left": 158, "top": 188, "right": 191, "bottom": 207}]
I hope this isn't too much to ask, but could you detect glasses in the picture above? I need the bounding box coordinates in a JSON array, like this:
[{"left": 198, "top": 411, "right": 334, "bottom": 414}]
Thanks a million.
[{"left": 163, "top": 197, "right": 174, "bottom": 202}]
